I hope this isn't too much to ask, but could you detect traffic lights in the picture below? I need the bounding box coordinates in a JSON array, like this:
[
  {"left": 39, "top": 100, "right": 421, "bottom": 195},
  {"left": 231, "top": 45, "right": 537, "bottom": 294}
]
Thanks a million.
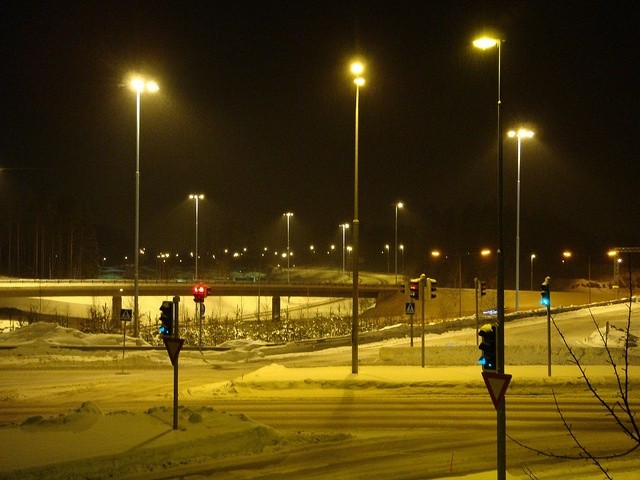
[
  {"left": 481, "top": 280, "right": 486, "bottom": 297},
  {"left": 478, "top": 326, "right": 496, "bottom": 370},
  {"left": 400, "top": 283, "right": 405, "bottom": 293},
  {"left": 193, "top": 285, "right": 208, "bottom": 303},
  {"left": 409, "top": 282, "right": 418, "bottom": 298},
  {"left": 430, "top": 279, "right": 437, "bottom": 298},
  {"left": 540, "top": 282, "right": 549, "bottom": 306},
  {"left": 158, "top": 301, "right": 174, "bottom": 337}
]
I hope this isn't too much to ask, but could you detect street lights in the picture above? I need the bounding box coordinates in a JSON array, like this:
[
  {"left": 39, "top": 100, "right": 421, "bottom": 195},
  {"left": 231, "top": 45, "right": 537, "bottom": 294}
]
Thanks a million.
[
  {"left": 399, "top": 245, "right": 404, "bottom": 275},
  {"left": 507, "top": 128, "right": 535, "bottom": 312},
  {"left": 339, "top": 224, "right": 349, "bottom": 286},
  {"left": 395, "top": 203, "right": 404, "bottom": 286},
  {"left": 385, "top": 244, "right": 390, "bottom": 275},
  {"left": 531, "top": 254, "right": 536, "bottom": 291},
  {"left": 473, "top": 37, "right": 505, "bottom": 480},
  {"left": 129, "top": 76, "right": 160, "bottom": 338},
  {"left": 189, "top": 194, "right": 204, "bottom": 281},
  {"left": 349, "top": 60, "right": 367, "bottom": 375},
  {"left": 283, "top": 213, "right": 294, "bottom": 284}
]
